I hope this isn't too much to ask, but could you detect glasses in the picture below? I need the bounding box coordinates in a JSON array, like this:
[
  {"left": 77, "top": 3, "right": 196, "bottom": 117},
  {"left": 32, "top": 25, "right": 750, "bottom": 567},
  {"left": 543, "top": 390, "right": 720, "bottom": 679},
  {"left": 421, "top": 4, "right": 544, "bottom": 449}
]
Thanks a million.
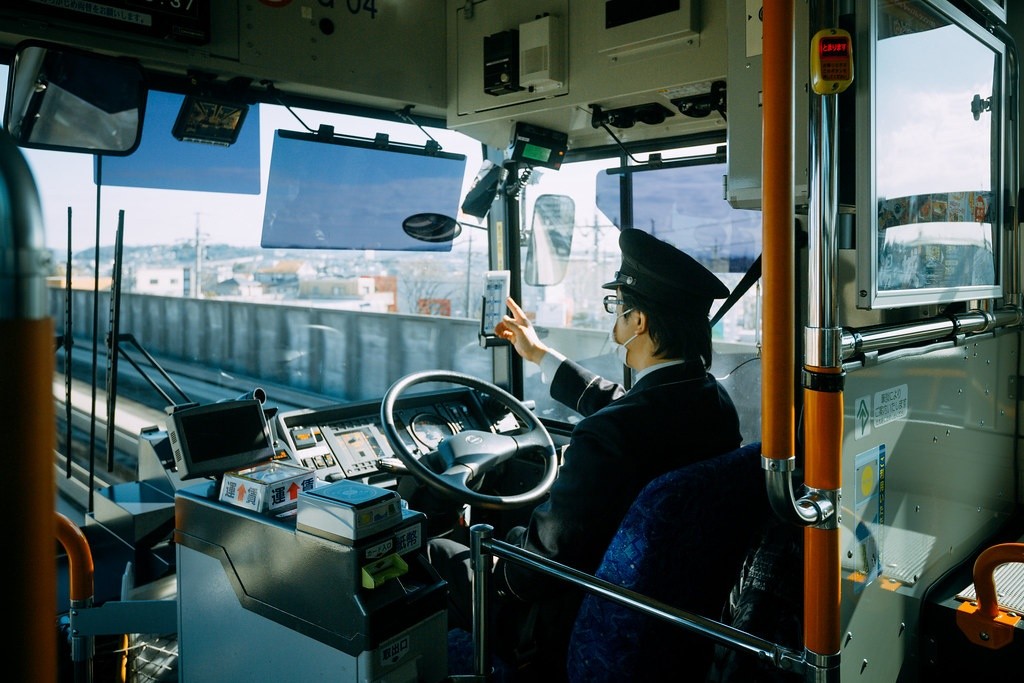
[{"left": 603, "top": 295, "right": 627, "bottom": 313}]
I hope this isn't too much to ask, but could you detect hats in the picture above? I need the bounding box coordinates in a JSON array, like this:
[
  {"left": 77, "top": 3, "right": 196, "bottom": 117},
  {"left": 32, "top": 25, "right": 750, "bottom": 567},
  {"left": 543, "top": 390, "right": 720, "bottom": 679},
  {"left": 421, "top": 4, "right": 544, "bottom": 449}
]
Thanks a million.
[{"left": 602, "top": 229, "right": 730, "bottom": 314}]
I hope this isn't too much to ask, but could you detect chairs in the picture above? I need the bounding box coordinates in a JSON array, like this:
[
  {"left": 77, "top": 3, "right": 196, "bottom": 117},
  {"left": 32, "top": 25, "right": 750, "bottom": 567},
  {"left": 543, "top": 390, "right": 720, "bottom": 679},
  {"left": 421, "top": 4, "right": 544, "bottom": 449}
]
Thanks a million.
[{"left": 447, "top": 440, "right": 761, "bottom": 683}]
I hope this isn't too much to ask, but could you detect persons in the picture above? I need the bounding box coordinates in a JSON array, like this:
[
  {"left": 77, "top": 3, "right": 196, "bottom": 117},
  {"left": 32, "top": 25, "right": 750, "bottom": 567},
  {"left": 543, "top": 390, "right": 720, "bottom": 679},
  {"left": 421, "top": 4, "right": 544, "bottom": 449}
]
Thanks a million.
[{"left": 420, "top": 229, "right": 742, "bottom": 683}]
